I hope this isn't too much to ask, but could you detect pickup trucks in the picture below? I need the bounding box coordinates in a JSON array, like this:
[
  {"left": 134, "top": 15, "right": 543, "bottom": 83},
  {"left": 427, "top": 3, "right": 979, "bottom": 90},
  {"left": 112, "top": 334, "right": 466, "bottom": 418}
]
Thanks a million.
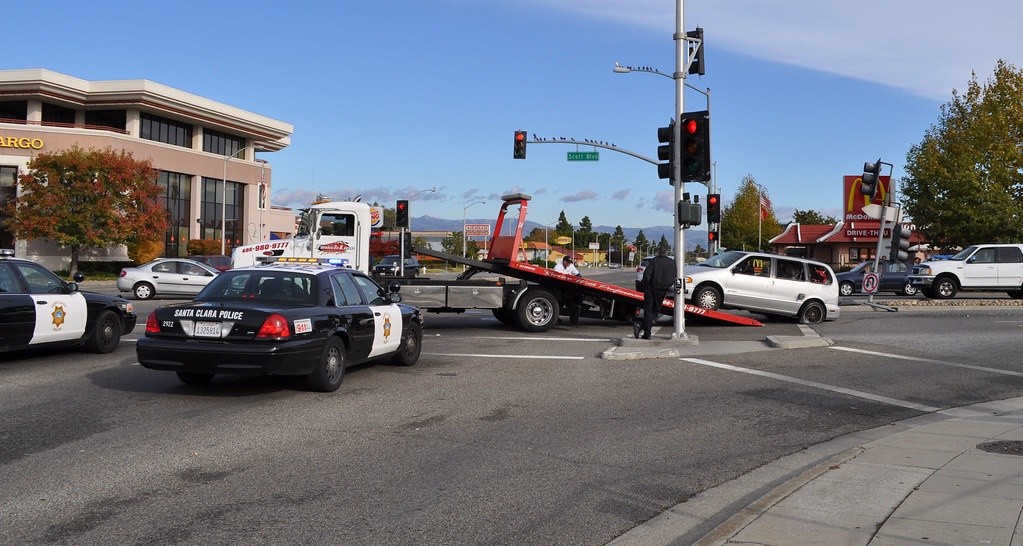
[
  {"left": 909, "top": 244, "right": 1023, "bottom": 299},
  {"left": 914, "top": 255, "right": 953, "bottom": 274}
]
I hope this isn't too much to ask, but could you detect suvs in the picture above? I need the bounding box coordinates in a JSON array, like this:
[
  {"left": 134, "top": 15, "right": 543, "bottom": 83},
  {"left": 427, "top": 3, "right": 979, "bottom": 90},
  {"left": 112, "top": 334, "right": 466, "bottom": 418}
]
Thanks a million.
[
  {"left": 372, "top": 253, "right": 421, "bottom": 277},
  {"left": 636, "top": 255, "right": 676, "bottom": 292}
]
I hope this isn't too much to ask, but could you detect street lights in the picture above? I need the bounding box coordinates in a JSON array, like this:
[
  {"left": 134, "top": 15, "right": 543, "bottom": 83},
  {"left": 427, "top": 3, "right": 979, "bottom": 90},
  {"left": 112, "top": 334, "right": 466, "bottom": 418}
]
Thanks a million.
[
  {"left": 463, "top": 201, "right": 486, "bottom": 272},
  {"left": 545, "top": 221, "right": 562, "bottom": 269},
  {"left": 612, "top": 63, "right": 712, "bottom": 262},
  {"left": 595, "top": 232, "right": 608, "bottom": 268},
  {"left": 622, "top": 239, "right": 657, "bottom": 268},
  {"left": 222, "top": 144, "right": 264, "bottom": 255},
  {"left": 509, "top": 212, "right": 529, "bottom": 236},
  {"left": 609, "top": 235, "right": 621, "bottom": 266},
  {"left": 573, "top": 227, "right": 586, "bottom": 266},
  {"left": 407, "top": 189, "right": 437, "bottom": 232}
]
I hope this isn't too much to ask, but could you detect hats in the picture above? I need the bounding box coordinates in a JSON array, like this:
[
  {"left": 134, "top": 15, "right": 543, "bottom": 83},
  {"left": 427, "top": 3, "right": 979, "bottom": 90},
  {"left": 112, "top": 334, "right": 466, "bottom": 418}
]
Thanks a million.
[
  {"left": 563, "top": 256, "right": 573, "bottom": 263},
  {"left": 658, "top": 247, "right": 667, "bottom": 254}
]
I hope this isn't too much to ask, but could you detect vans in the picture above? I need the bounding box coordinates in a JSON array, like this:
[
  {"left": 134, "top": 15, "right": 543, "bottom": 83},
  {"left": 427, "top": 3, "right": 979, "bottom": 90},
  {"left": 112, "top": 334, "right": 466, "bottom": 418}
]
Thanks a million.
[{"left": 666, "top": 250, "right": 842, "bottom": 324}]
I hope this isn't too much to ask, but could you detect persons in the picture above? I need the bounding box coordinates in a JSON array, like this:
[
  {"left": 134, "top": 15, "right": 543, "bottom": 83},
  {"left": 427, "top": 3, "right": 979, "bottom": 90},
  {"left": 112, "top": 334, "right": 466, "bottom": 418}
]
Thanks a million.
[
  {"left": 710, "top": 252, "right": 922, "bottom": 280},
  {"left": 552, "top": 255, "right": 582, "bottom": 278},
  {"left": 183, "top": 265, "right": 204, "bottom": 277},
  {"left": 632, "top": 246, "right": 677, "bottom": 341}
]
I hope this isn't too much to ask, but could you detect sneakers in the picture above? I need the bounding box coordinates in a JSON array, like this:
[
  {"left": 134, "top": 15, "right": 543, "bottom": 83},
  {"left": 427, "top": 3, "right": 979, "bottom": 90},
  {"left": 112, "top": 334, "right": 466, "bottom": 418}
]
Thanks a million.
[
  {"left": 641, "top": 331, "right": 651, "bottom": 339},
  {"left": 632, "top": 322, "right": 640, "bottom": 339}
]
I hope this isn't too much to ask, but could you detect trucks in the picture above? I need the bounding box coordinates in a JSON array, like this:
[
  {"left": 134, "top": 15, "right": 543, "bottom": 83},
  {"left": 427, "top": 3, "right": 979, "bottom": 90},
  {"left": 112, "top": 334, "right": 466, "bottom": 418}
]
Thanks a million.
[{"left": 232, "top": 194, "right": 763, "bottom": 332}]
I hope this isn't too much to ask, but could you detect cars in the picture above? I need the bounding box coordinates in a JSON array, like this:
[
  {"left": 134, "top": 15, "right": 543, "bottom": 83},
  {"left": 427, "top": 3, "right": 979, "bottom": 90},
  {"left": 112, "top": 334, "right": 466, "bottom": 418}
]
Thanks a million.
[
  {"left": 117, "top": 257, "right": 239, "bottom": 301},
  {"left": 0, "top": 248, "right": 137, "bottom": 356},
  {"left": 186, "top": 254, "right": 233, "bottom": 275},
  {"left": 835, "top": 261, "right": 918, "bottom": 297},
  {"left": 135, "top": 255, "right": 424, "bottom": 393}
]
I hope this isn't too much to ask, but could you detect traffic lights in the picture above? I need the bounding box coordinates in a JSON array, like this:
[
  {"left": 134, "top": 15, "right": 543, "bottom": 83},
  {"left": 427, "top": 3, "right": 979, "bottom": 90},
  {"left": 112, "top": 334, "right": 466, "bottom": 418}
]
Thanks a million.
[
  {"left": 680, "top": 111, "right": 709, "bottom": 181},
  {"left": 860, "top": 158, "right": 882, "bottom": 197},
  {"left": 708, "top": 232, "right": 718, "bottom": 242},
  {"left": 707, "top": 194, "right": 720, "bottom": 224},
  {"left": 890, "top": 225, "right": 911, "bottom": 261},
  {"left": 658, "top": 117, "right": 676, "bottom": 185},
  {"left": 513, "top": 130, "right": 526, "bottom": 160},
  {"left": 396, "top": 199, "right": 408, "bottom": 227}
]
